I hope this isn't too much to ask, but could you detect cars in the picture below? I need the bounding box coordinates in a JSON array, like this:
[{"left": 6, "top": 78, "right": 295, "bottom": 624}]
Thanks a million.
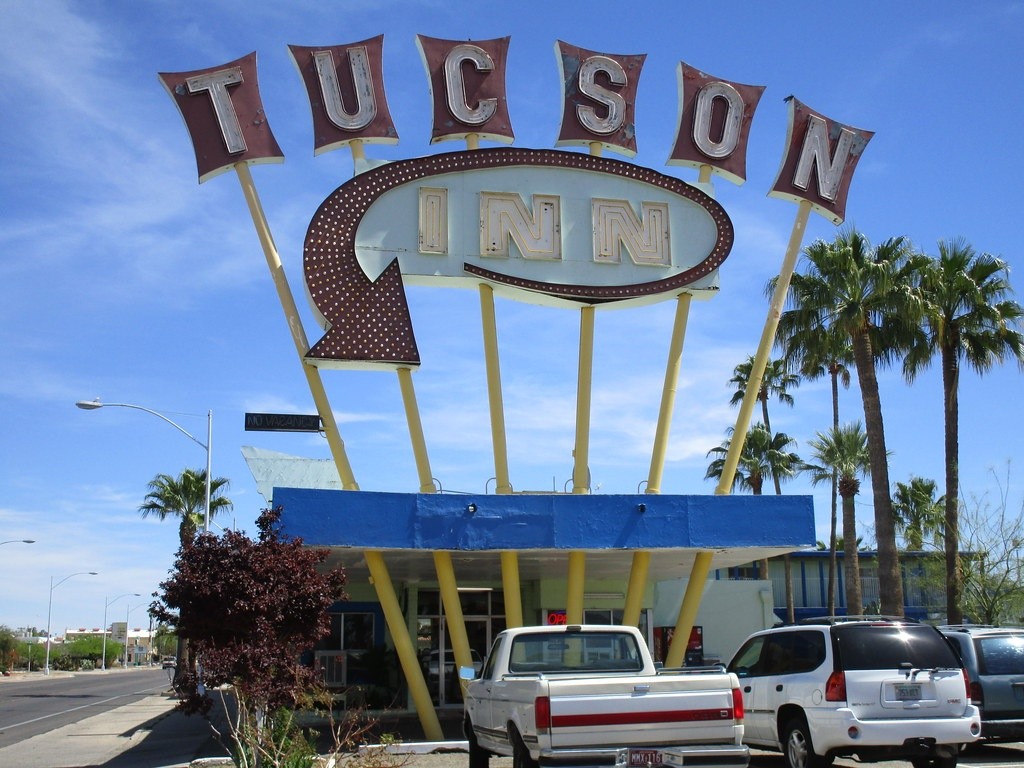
[
  {"left": 729, "top": 614, "right": 981, "bottom": 768},
  {"left": 162, "top": 656, "right": 178, "bottom": 670},
  {"left": 425, "top": 648, "right": 484, "bottom": 694},
  {"left": 935, "top": 623, "right": 1024, "bottom": 751}
]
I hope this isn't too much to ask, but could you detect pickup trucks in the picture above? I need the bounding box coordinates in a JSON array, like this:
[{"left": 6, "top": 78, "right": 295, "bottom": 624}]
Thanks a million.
[{"left": 461, "top": 624, "right": 751, "bottom": 768}]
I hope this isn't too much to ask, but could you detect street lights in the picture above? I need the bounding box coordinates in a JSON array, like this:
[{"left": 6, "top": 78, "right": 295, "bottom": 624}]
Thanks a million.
[
  {"left": 75, "top": 396, "right": 214, "bottom": 550},
  {"left": 101, "top": 593, "right": 142, "bottom": 671},
  {"left": 123, "top": 601, "right": 154, "bottom": 669},
  {"left": 42, "top": 571, "right": 99, "bottom": 675}
]
